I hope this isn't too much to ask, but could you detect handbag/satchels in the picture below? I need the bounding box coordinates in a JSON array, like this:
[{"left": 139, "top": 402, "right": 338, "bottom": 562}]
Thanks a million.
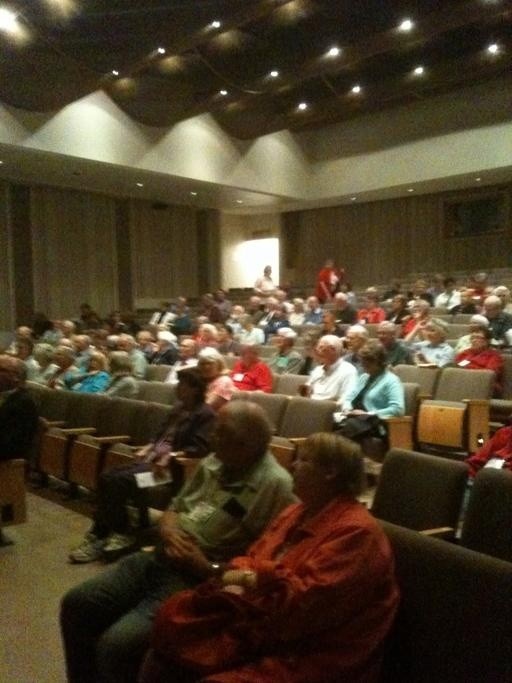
[{"left": 148, "top": 580, "right": 270, "bottom": 672}]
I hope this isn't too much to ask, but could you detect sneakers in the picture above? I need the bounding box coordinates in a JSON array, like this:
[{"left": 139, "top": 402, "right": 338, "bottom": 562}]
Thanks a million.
[
  {"left": 103, "top": 529, "right": 138, "bottom": 552},
  {"left": 67, "top": 532, "right": 106, "bottom": 562}
]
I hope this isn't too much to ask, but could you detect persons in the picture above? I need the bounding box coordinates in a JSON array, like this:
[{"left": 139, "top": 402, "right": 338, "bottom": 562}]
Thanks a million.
[{"left": 0, "top": 260, "right": 512, "bottom": 683}]
